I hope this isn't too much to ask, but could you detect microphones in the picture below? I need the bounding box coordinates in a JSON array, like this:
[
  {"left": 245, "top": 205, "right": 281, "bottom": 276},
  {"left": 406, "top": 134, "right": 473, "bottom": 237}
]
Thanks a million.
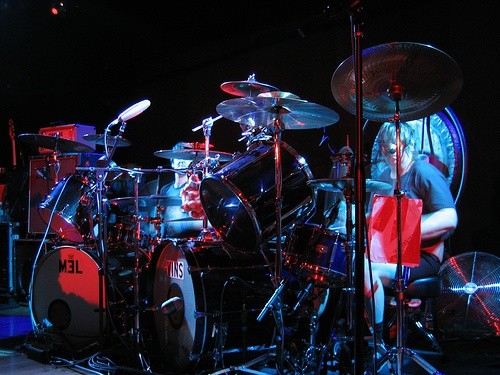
[{"left": 107, "top": 99, "right": 151, "bottom": 126}]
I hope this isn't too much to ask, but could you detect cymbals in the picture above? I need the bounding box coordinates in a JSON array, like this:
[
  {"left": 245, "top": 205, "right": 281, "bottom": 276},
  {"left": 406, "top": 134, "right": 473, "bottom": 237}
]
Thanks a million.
[
  {"left": 329, "top": 40, "right": 464, "bottom": 124},
  {"left": 153, "top": 149, "right": 234, "bottom": 164},
  {"left": 216, "top": 80, "right": 340, "bottom": 134},
  {"left": 305, "top": 174, "right": 393, "bottom": 193},
  {"left": 82, "top": 133, "right": 131, "bottom": 149},
  {"left": 18, "top": 133, "right": 97, "bottom": 152}
]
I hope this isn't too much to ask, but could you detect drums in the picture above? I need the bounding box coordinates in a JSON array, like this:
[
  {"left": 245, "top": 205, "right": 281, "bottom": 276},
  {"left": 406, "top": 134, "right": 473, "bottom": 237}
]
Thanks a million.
[
  {"left": 35, "top": 172, "right": 112, "bottom": 249},
  {"left": 280, "top": 221, "right": 367, "bottom": 286},
  {"left": 199, "top": 139, "right": 319, "bottom": 251},
  {"left": 27, "top": 243, "right": 151, "bottom": 355},
  {"left": 145, "top": 238, "right": 276, "bottom": 375}
]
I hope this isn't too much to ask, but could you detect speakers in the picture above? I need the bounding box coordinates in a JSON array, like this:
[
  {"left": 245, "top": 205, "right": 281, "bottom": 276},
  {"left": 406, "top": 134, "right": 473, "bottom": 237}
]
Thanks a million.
[{"left": 28, "top": 152, "right": 103, "bottom": 235}]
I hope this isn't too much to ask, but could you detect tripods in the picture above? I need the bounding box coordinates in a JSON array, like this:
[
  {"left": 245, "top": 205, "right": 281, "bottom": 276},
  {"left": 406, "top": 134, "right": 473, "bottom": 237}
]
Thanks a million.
[{"left": 49, "top": 21, "right": 440, "bottom": 375}]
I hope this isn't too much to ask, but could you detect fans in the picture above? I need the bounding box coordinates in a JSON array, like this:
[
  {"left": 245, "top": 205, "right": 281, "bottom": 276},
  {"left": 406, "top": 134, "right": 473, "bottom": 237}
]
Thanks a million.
[{"left": 436, "top": 251, "right": 500, "bottom": 341}]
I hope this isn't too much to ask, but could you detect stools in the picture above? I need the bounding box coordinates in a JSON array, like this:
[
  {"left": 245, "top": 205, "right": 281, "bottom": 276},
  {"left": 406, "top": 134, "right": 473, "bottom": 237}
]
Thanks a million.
[{"left": 383, "top": 277, "right": 445, "bottom": 353}]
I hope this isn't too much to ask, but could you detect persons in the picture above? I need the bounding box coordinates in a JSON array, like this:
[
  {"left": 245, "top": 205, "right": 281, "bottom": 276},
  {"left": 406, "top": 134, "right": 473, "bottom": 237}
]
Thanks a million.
[
  {"left": 353, "top": 122, "right": 458, "bottom": 357},
  {"left": 158, "top": 143, "right": 213, "bottom": 240}
]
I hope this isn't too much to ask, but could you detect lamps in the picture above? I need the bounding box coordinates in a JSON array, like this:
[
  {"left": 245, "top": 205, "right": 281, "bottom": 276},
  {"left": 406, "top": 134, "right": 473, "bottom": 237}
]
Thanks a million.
[{"left": 48, "top": 0, "right": 79, "bottom": 19}]
